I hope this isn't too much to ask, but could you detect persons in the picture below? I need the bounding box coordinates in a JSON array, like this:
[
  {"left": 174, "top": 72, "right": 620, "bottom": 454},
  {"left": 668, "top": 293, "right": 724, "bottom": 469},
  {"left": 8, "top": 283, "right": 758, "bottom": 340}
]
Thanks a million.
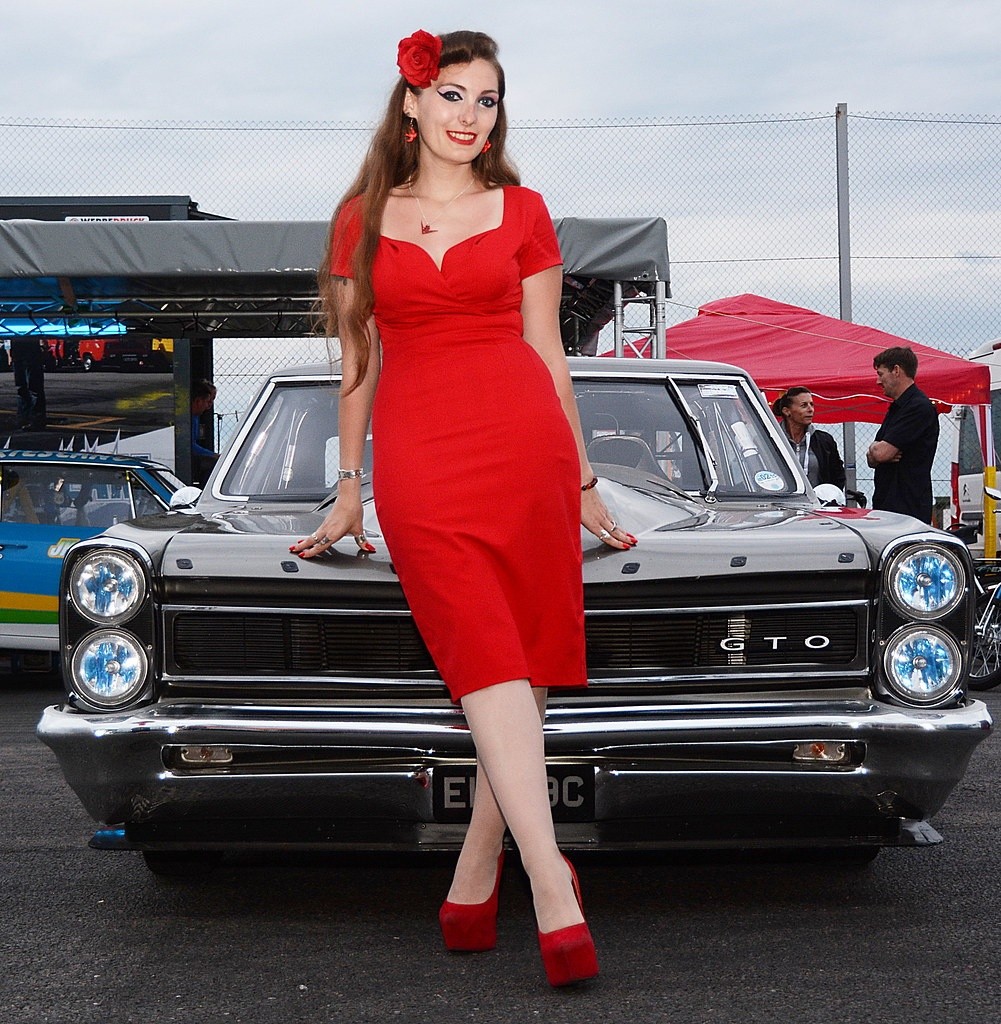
[
  {"left": 773, "top": 387, "right": 847, "bottom": 493},
  {"left": 866, "top": 347, "right": 941, "bottom": 527},
  {"left": 289, "top": 30, "right": 619, "bottom": 990}
]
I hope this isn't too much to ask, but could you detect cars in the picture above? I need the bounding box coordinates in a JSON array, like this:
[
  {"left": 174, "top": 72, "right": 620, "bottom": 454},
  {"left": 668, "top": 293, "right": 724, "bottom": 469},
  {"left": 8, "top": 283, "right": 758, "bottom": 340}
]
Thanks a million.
[
  {"left": 0, "top": 330, "right": 163, "bottom": 373},
  {"left": 33, "top": 352, "right": 996, "bottom": 898}
]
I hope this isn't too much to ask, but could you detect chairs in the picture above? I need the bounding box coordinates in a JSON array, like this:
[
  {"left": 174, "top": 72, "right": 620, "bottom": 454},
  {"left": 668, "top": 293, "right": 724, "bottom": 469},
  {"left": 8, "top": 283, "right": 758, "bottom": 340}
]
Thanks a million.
[{"left": 586, "top": 435, "right": 670, "bottom": 484}]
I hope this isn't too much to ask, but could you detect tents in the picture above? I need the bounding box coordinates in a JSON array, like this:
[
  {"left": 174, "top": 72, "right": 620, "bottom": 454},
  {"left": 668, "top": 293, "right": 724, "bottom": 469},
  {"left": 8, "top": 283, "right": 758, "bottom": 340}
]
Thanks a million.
[{"left": 596, "top": 292, "right": 996, "bottom": 558}]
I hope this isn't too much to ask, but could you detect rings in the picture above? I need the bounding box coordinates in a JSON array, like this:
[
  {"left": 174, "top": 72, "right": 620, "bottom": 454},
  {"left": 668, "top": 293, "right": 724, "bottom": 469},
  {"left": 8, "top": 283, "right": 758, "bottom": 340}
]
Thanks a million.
[
  {"left": 354, "top": 533, "right": 367, "bottom": 544},
  {"left": 312, "top": 532, "right": 320, "bottom": 543},
  {"left": 608, "top": 521, "right": 618, "bottom": 533},
  {"left": 599, "top": 530, "right": 610, "bottom": 540},
  {"left": 320, "top": 536, "right": 332, "bottom": 546}
]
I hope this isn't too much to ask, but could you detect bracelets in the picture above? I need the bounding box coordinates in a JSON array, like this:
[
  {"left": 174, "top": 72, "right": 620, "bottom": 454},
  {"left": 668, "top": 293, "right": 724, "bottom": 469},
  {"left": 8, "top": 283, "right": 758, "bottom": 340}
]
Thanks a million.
[
  {"left": 337, "top": 466, "right": 363, "bottom": 481},
  {"left": 581, "top": 477, "right": 598, "bottom": 491}
]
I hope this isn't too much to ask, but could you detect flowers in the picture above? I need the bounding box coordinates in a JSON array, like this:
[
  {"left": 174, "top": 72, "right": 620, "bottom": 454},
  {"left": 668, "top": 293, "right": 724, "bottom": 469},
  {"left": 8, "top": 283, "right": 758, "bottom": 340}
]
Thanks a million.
[{"left": 397, "top": 28, "right": 442, "bottom": 90}]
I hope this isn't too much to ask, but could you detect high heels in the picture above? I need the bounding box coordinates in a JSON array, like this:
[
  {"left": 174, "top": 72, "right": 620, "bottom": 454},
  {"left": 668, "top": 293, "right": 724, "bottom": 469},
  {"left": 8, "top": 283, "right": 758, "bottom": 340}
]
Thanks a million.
[
  {"left": 439, "top": 842, "right": 504, "bottom": 950},
  {"left": 538, "top": 852, "right": 600, "bottom": 985}
]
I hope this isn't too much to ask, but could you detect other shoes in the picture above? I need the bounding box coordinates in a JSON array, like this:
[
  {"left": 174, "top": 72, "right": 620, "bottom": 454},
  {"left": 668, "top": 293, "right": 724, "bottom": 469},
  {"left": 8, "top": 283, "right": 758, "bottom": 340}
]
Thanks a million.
[
  {"left": 22, "top": 422, "right": 48, "bottom": 431},
  {"left": 7, "top": 418, "right": 32, "bottom": 431}
]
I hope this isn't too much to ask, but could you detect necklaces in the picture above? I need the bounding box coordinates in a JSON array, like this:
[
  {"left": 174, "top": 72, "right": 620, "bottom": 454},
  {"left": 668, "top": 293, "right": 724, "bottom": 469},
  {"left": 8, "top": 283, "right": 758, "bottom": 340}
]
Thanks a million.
[{"left": 408, "top": 170, "right": 477, "bottom": 234}]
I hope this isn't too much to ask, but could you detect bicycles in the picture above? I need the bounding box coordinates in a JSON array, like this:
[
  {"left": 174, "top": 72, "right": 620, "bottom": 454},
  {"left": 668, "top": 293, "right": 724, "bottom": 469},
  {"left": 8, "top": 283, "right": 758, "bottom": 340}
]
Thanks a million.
[{"left": 942, "top": 523, "right": 1001, "bottom": 691}]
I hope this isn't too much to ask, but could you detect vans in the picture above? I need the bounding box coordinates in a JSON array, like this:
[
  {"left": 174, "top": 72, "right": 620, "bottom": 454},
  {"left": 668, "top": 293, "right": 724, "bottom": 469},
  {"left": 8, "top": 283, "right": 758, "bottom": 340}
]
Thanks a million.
[{"left": 0, "top": 447, "right": 197, "bottom": 676}]
